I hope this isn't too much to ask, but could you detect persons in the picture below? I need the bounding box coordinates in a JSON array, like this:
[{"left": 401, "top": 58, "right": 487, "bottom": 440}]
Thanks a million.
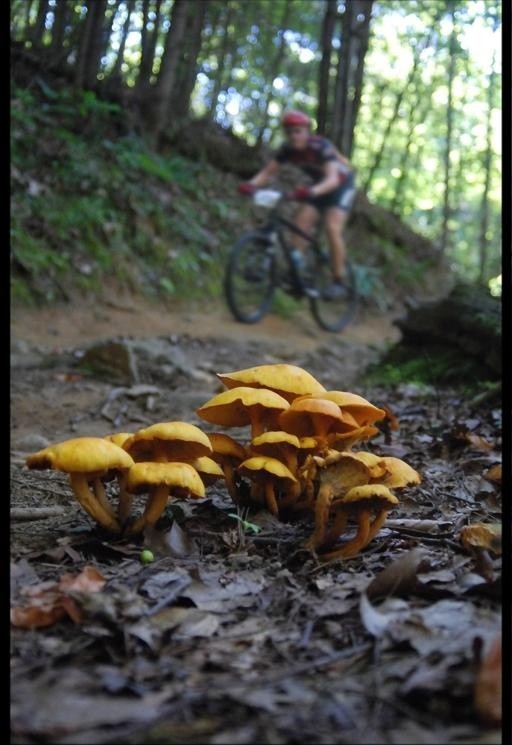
[{"left": 239, "top": 111, "right": 358, "bottom": 302}]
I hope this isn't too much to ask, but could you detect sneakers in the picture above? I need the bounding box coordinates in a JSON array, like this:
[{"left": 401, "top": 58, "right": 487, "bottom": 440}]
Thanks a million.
[{"left": 323, "top": 283, "right": 352, "bottom": 299}]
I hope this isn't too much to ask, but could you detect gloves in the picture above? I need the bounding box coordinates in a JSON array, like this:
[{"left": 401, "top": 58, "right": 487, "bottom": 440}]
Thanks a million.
[
  {"left": 295, "top": 185, "right": 310, "bottom": 199},
  {"left": 238, "top": 182, "right": 253, "bottom": 194}
]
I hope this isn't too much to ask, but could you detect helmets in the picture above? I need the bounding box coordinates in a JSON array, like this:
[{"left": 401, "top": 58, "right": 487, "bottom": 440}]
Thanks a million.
[{"left": 284, "top": 112, "right": 309, "bottom": 125}]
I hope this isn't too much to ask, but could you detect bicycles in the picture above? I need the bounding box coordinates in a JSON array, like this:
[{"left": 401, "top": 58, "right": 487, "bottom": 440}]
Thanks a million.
[{"left": 224, "top": 184, "right": 361, "bottom": 333}]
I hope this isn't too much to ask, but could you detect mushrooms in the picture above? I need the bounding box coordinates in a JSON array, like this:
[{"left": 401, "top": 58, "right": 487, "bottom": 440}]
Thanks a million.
[{"left": 26, "top": 364, "right": 421, "bottom": 560}]
